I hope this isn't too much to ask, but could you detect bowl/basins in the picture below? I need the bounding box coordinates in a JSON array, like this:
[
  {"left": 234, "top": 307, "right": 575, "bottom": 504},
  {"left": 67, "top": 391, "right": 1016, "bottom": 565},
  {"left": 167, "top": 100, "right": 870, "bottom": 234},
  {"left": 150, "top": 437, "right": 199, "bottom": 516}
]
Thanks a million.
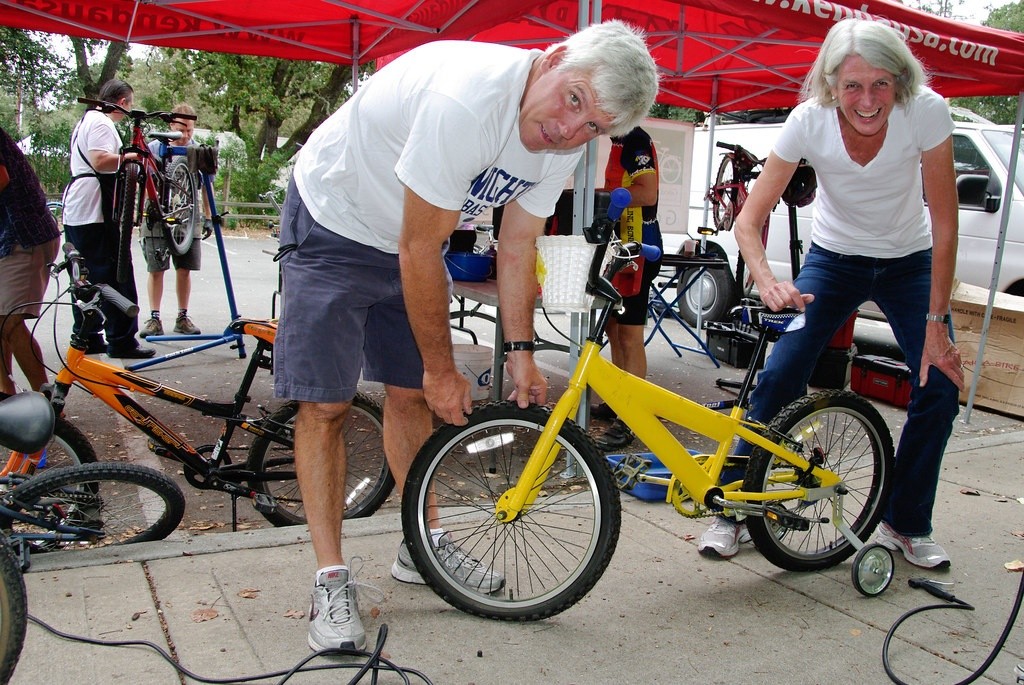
[{"left": 443, "top": 252, "right": 494, "bottom": 282}]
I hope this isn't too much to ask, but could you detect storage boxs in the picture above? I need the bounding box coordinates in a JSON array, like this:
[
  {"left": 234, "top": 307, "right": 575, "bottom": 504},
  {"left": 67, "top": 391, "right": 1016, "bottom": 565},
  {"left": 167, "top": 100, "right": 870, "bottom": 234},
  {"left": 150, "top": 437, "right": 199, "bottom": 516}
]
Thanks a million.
[
  {"left": 951, "top": 283, "right": 1024, "bottom": 417},
  {"left": 851, "top": 354, "right": 914, "bottom": 408},
  {"left": 808, "top": 309, "right": 859, "bottom": 391},
  {"left": 706, "top": 328, "right": 768, "bottom": 369},
  {"left": 606, "top": 449, "right": 703, "bottom": 502}
]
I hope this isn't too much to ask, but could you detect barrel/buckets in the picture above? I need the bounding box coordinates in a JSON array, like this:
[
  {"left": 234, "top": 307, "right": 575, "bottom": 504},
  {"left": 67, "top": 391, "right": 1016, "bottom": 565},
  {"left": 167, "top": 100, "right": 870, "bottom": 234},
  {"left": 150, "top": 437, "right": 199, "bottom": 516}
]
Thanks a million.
[
  {"left": 827, "top": 308, "right": 859, "bottom": 349},
  {"left": 452, "top": 344, "right": 494, "bottom": 400}
]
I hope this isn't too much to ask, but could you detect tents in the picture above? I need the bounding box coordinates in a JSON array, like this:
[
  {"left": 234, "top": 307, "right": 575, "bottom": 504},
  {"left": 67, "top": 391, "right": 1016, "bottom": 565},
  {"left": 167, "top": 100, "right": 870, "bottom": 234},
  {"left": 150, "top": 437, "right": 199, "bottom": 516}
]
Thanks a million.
[{"left": 0, "top": 0, "right": 1024, "bottom": 426}]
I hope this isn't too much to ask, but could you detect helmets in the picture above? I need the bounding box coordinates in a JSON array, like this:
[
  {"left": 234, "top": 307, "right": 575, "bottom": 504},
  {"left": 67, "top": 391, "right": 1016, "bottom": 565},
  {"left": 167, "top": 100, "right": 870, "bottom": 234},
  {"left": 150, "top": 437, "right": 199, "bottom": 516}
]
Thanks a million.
[{"left": 780, "top": 162, "right": 817, "bottom": 208}]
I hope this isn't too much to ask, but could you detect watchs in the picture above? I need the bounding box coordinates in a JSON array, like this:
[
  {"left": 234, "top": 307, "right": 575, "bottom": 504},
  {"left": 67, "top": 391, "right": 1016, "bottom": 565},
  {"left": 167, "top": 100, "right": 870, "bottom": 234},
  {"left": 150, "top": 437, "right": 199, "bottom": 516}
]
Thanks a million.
[{"left": 926, "top": 313, "right": 950, "bottom": 326}]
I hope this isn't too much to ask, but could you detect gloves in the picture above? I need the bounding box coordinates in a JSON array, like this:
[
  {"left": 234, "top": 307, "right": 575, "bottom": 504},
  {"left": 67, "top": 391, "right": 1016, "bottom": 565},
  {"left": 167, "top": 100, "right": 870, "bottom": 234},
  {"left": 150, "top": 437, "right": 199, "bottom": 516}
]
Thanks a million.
[{"left": 201, "top": 218, "right": 212, "bottom": 239}]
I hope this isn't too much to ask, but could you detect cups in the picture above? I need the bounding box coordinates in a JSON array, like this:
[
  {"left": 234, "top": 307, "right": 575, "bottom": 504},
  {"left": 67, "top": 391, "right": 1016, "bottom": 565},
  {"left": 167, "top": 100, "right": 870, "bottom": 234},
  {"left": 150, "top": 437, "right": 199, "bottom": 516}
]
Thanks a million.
[{"left": 684, "top": 239, "right": 697, "bottom": 256}]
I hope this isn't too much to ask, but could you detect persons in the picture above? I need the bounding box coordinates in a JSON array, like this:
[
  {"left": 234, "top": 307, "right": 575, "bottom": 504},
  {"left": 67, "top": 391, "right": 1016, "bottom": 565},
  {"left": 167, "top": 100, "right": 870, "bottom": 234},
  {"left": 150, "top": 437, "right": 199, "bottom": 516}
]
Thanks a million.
[
  {"left": 589, "top": 124, "right": 664, "bottom": 451},
  {"left": 60, "top": 79, "right": 156, "bottom": 358},
  {"left": 272, "top": 21, "right": 661, "bottom": 655},
  {"left": 0, "top": 124, "right": 60, "bottom": 408},
  {"left": 139, "top": 105, "right": 215, "bottom": 338},
  {"left": 696, "top": 18, "right": 964, "bottom": 570}
]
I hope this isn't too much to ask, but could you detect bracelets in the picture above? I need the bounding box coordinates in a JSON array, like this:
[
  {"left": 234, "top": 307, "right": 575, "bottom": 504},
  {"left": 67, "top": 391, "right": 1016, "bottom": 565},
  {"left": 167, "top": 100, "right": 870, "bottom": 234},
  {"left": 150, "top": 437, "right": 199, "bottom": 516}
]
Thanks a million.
[{"left": 503, "top": 340, "right": 536, "bottom": 355}]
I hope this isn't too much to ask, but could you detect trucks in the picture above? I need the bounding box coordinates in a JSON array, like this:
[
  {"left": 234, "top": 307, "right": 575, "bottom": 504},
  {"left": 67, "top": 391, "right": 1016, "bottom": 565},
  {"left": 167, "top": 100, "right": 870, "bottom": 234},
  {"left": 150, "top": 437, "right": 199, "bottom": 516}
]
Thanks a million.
[{"left": 689, "top": 99, "right": 1024, "bottom": 325}]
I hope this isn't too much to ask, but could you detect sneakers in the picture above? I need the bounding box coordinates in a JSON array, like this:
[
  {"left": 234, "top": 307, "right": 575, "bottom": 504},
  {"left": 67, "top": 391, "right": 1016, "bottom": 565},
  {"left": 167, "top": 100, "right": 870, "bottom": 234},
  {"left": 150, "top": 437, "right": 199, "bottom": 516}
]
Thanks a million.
[
  {"left": 108, "top": 342, "right": 155, "bottom": 357},
  {"left": 86, "top": 340, "right": 107, "bottom": 354},
  {"left": 698, "top": 514, "right": 751, "bottom": 557},
  {"left": 589, "top": 400, "right": 617, "bottom": 420},
  {"left": 175, "top": 315, "right": 201, "bottom": 335},
  {"left": 876, "top": 521, "right": 951, "bottom": 569},
  {"left": 392, "top": 534, "right": 506, "bottom": 594},
  {"left": 139, "top": 318, "right": 164, "bottom": 338},
  {"left": 305, "top": 556, "right": 386, "bottom": 654},
  {"left": 596, "top": 418, "right": 634, "bottom": 451}
]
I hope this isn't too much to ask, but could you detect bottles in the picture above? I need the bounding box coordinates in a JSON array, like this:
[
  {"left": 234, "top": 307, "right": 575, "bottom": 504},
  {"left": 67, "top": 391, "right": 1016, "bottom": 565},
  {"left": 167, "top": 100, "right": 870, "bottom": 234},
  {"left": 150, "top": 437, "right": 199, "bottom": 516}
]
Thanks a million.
[{"left": 486, "top": 245, "right": 497, "bottom": 281}]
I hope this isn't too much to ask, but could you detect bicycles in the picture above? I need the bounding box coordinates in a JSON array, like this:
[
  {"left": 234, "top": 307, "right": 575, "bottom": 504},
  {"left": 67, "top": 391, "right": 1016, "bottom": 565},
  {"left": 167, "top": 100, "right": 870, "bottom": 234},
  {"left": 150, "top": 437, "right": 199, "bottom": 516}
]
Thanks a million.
[
  {"left": 399, "top": 186, "right": 895, "bottom": 622},
  {"left": 0, "top": 241, "right": 399, "bottom": 535},
  {"left": 0, "top": 532, "right": 28, "bottom": 685},
  {"left": 0, "top": 391, "right": 186, "bottom": 554},
  {"left": 77, "top": 97, "right": 198, "bottom": 282}
]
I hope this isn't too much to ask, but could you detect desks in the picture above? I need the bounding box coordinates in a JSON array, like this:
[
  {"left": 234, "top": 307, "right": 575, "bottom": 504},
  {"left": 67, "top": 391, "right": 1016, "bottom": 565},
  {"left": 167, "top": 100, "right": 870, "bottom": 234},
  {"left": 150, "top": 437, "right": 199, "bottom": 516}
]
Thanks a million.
[
  {"left": 644, "top": 259, "right": 729, "bottom": 368},
  {"left": 450, "top": 279, "right": 597, "bottom": 430}
]
